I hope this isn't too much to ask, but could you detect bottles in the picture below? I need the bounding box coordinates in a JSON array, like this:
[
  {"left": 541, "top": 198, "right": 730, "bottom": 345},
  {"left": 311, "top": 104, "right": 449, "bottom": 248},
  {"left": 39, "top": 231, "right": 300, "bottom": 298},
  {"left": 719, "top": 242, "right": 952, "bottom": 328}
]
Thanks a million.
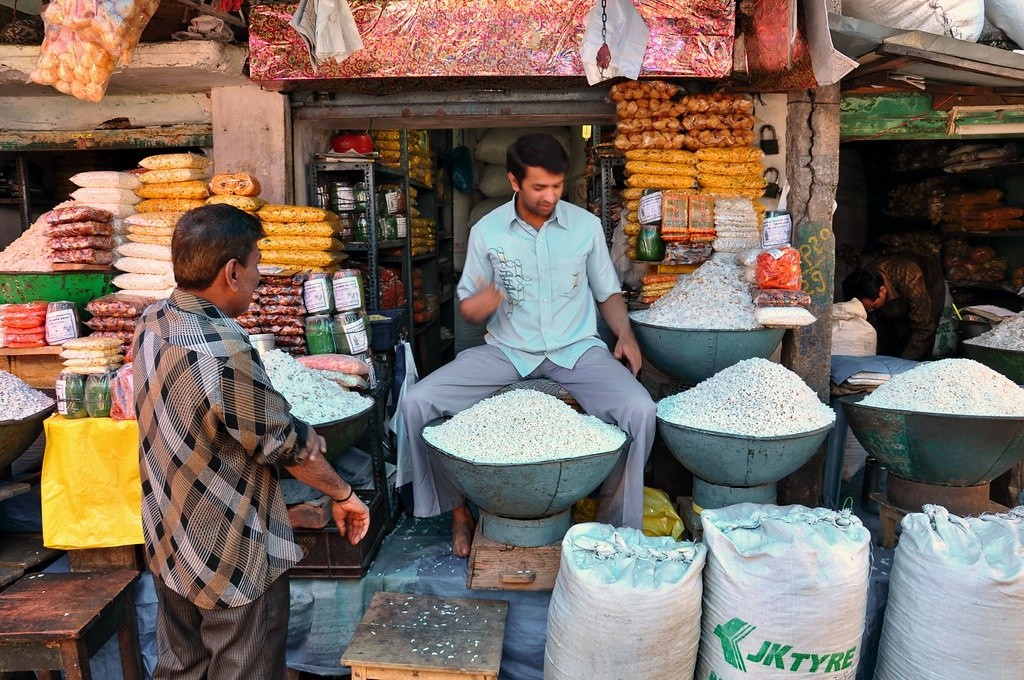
[{"left": 635, "top": 225, "right": 663, "bottom": 261}]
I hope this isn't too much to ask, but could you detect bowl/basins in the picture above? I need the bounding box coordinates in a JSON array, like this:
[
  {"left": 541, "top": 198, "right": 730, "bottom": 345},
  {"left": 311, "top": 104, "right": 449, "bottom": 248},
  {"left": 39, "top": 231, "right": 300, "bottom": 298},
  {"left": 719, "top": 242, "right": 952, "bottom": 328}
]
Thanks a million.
[
  {"left": 307, "top": 394, "right": 375, "bottom": 462},
  {"left": 0, "top": 402, "right": 56, "bottom": 469},
  {"left": 419, "top": 308, "right": 1024, "bottom": 520}
]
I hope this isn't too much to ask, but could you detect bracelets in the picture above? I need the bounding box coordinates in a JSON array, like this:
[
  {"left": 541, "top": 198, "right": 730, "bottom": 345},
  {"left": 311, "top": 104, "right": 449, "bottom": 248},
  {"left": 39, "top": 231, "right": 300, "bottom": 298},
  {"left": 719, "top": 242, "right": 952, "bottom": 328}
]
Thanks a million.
[{"left": 331, "top": 488, "right": 354, "bottom": 502}]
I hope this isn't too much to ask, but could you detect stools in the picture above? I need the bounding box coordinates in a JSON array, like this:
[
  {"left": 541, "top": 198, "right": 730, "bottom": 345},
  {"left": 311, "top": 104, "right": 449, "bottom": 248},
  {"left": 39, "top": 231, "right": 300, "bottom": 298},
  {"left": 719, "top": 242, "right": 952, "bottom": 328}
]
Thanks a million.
[
  {"left": 0, "top": 568, "right": 144, "bottom": 680},
  {"left": 497, "top": 377, "right": 579, "bottom": 411},
  {"left": 339, "top": 591, "right": 509, "bottom": 680}
]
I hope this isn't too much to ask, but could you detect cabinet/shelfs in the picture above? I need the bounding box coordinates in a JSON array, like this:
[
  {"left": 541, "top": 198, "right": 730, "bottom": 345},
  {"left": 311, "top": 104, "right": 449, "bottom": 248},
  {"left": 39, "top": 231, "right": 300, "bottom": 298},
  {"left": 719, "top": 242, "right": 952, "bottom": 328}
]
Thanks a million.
[{"left": 309, "top": 157, "right": 439, "bottom": 380}]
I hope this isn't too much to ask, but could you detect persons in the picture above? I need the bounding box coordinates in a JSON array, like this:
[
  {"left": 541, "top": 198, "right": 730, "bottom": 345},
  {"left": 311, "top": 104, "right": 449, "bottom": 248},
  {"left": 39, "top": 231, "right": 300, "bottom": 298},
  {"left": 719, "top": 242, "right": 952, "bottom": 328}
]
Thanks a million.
[
  {"left": 400, "top": 133, "right": 659, "bottom": 558},
  {"left": 131, "top": 205, "right": 370, "bottom": 679}
]
